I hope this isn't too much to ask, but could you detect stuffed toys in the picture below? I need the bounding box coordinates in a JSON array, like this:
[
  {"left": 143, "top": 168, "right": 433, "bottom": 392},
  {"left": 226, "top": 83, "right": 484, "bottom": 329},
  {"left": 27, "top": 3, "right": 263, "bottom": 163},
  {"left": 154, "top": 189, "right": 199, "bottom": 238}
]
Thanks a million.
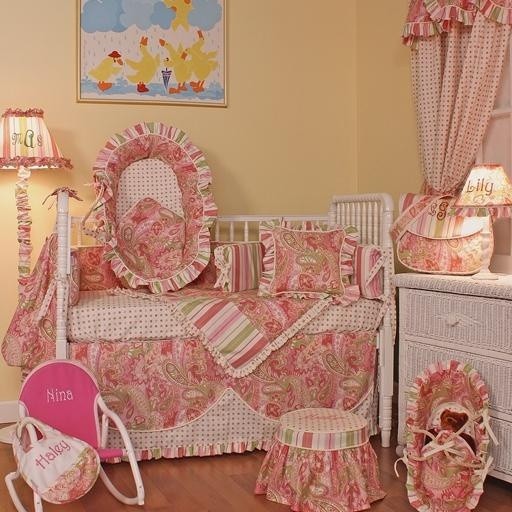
[{"left": 424, "top": 409, "right": 476, "bottom": 455}]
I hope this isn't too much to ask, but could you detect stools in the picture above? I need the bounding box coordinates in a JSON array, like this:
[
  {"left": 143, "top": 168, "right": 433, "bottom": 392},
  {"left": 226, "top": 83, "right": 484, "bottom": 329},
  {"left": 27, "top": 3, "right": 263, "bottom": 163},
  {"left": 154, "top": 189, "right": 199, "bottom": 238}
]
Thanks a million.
[{"left": 255, "top": 407, "right": 387, "bottom": 512}]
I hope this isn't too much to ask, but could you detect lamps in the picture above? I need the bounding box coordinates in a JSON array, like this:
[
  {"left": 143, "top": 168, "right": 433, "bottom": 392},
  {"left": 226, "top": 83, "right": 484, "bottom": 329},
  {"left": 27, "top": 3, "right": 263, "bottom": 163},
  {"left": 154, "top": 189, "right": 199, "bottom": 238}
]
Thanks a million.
[
  {"left": 1, "top": 106, "right": 73, "bottom": 445},
  {"left": 449, "top": 164, "right": 512, "bottom": 281}
]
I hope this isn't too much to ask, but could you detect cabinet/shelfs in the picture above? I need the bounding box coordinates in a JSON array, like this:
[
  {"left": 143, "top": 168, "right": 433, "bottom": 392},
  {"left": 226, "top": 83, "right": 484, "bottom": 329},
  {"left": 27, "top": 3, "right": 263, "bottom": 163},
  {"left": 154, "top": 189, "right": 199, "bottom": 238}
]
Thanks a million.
[{"left": 396, "top": 271, "right": 512, "bottom": 485}]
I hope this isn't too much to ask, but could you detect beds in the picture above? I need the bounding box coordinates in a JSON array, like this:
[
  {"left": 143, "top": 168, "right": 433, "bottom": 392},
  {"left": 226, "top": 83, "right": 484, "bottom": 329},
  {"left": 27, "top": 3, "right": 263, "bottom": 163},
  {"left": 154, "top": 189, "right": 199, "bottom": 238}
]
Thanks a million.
[{"left": 55, "top": 189, "right": 395, "bottom": 464}]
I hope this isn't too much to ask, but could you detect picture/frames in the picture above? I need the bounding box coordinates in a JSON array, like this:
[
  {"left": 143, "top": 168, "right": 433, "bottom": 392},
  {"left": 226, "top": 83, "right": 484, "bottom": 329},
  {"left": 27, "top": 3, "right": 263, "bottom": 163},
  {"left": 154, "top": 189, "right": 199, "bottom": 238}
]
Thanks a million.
[{"left": 75, "top": 0, "right": 228, "bottom": 107}]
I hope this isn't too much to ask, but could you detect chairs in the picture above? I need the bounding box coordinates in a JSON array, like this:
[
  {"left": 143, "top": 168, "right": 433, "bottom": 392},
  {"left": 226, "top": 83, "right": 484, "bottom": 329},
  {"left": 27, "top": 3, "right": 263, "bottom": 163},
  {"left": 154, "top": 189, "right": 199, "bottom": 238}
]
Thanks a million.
[{"left": 4, "top": 359, "right": 145, "bottom": 512}]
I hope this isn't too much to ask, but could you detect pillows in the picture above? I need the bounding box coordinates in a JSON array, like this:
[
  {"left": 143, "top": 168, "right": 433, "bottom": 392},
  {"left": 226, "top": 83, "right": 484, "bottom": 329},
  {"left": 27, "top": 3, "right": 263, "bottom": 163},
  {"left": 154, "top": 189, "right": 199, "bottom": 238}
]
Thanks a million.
[{"left": 257, "top": 218, "right": 360, "bottom": 307}]
